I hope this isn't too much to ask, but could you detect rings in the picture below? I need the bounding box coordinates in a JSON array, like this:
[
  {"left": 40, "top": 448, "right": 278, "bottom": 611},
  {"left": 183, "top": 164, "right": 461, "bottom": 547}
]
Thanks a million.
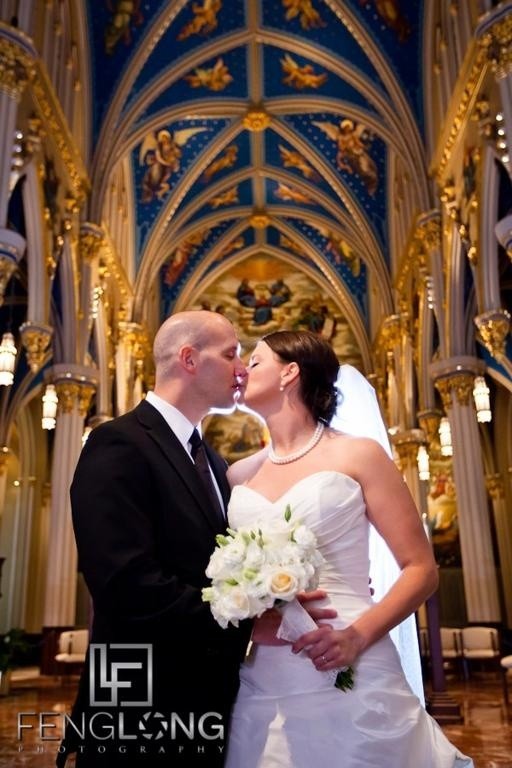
[{"left": 321, "top": 654, "right": 328, "bottom": 663}]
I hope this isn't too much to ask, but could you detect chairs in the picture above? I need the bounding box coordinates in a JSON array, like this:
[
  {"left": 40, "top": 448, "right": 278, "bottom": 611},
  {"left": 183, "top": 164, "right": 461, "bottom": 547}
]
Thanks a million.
[
  {"left": 416, "top": 626, "right": 512, "bottom": 708},
  {"left": 52, "top": 629, "right": 92, "bottom": 680}
]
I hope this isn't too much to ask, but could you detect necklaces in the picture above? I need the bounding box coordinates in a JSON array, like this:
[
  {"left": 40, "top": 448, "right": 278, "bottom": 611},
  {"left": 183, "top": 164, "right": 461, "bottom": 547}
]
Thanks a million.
[{"left": 264, "top": 418, "right": 324, "bottom": 464}]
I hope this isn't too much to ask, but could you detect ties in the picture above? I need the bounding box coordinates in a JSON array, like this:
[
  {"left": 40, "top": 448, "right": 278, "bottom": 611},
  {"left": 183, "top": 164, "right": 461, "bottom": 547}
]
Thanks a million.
[{"left": 190, "top": 430, "right": 224, "bottom": 522}]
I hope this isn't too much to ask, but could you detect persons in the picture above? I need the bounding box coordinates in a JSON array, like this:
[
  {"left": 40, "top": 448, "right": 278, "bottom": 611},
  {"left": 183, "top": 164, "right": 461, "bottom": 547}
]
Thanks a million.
[
  {"left": 55, "top": 309, "right": 341, "bottom": 768},
  {"left": 219, "top": 329, "right": 472, "bottom": 768}
]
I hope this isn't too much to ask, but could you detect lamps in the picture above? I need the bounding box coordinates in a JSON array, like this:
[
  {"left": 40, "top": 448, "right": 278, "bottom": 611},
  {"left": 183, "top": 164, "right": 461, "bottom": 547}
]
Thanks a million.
[
  {"left": 41, "top": 360, "right": 59, "bottom": 430},
  {"left": 438, "top": 414, "right": 455, "bottom": 459},
  {"left": 414, "top": 444, "right": 432, "bottom": 483},
  {"left": 470, "top": 337, "right": 494, "bottom": 424},
  {"left": 0, "top": 273, "right": 21, "bottom": 386}
]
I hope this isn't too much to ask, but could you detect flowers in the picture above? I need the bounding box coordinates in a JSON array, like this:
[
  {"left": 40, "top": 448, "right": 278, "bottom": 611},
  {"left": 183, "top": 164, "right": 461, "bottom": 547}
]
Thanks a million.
[{"left": 188, "top": 497, "right": 361, "bottom": 698}]
